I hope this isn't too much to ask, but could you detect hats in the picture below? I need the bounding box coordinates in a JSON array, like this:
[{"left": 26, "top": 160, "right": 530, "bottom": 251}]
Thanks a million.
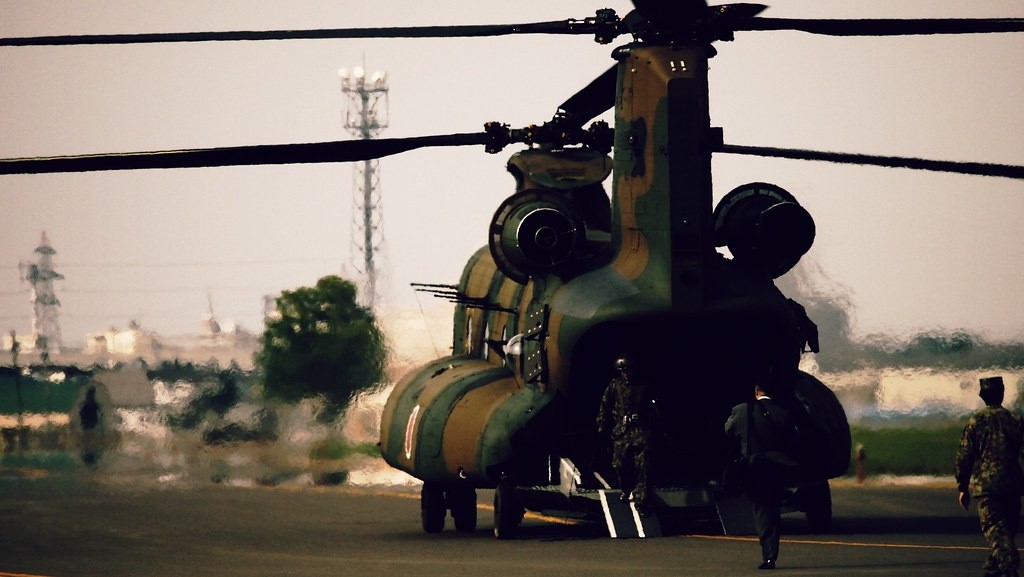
[{"left": 979, "top": 376, "right": 1003, "bottom": 390}]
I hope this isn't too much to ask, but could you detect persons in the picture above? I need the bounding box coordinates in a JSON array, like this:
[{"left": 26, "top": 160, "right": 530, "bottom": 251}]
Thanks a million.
[
  {"left": 594, "top": 352, "right": 656, "bottom": 516},
  {"left": 955, "top": 377, "right": 1024, "bottom": 577},
  {"left": 724, "top": 372, "right": 795, "bottom": 569}
]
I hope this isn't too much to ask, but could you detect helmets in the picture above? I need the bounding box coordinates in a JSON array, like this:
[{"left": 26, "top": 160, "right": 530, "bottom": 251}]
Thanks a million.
[{"left": 616, "top": 357, "right": 635, "bottom": 371}]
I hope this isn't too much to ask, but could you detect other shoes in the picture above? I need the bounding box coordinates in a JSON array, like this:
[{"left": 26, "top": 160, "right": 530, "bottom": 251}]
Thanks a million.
[
  {"left": 984, "top": 572, "right": 1020, "bottom": 577},
  {"left": 619, "top": 492, "right": 628, "bottom": 502},
  {"left": 636, "top": 504, "right": 648, "bottom": 517}
]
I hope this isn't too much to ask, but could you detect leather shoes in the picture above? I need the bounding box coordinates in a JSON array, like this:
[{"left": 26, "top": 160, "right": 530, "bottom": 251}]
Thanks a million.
[{"left": 758, "top": 559, "right": 776, "bottom": 570}]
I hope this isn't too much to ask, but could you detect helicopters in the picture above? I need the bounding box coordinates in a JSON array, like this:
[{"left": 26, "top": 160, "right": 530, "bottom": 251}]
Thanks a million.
[{"left": 1, "top": 0, "right": 1024, "bottom": 566}]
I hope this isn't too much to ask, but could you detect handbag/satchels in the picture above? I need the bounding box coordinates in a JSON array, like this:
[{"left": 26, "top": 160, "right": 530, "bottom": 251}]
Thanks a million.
[{"left": 722, "top": 455, "right": 753, "bottom": 492}]
